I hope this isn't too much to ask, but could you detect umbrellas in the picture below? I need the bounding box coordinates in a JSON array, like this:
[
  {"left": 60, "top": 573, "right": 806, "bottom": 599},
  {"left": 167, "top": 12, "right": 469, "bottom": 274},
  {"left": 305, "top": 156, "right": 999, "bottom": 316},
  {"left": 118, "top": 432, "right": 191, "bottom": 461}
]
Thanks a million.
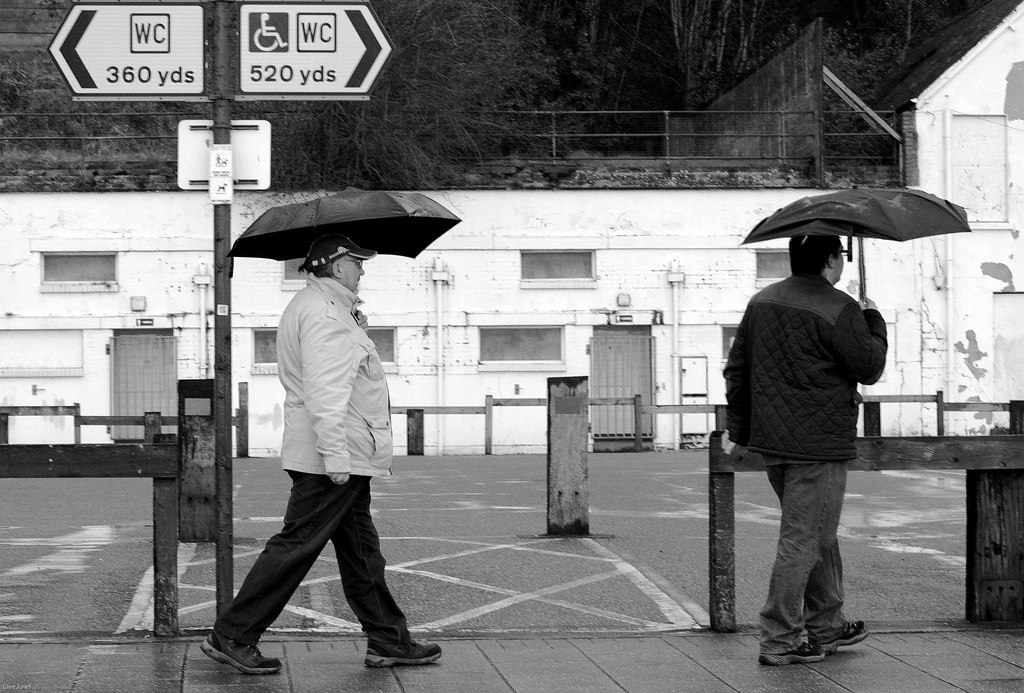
[
  {"left": 224, "top": 181, "right": 459, "bottom": 261},
  {"left": 738, "top": 183, "right": 973, "bottom": 306}
]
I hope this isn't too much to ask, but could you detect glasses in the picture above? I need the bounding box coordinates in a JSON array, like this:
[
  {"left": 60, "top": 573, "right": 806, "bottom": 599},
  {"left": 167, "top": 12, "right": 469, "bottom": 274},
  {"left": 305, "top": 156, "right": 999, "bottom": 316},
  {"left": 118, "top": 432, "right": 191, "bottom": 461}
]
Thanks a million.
[
  {"left": 345, "top": 259, "right": 363, "bottom": 269},
  {"left": 832, "top": 248, "right": 851, "bottom": 257}
]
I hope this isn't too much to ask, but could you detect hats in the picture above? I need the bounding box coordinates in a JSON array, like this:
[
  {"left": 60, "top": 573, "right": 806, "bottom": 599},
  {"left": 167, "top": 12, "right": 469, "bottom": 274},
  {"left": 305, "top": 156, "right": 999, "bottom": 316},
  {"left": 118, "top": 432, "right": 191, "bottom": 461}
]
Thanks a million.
[{"left": 307, "top": 234, "right": 377, "bottom": 266}]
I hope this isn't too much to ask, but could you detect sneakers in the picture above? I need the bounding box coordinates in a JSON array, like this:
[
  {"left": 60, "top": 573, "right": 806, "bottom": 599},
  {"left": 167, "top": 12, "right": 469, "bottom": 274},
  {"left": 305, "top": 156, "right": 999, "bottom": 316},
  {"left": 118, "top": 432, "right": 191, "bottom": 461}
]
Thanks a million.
[
  {"left": 364, "top": 638, "right": 441, "bottom": 668},
  {"left": 808, "top": 621, "right": 869, "bottom": 654},
  {"left": 759, "top": 642, "right": 825, "bottom": 665},
  {"left": 200, "top": 626, "right": 282, "bottom": 674}
]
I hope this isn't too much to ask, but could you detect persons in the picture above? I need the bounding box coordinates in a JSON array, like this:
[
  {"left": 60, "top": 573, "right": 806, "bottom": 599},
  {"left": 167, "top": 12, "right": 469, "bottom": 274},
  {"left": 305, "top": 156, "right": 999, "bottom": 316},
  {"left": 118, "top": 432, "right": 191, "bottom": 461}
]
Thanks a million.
[
  {"left": 199, "top": 221, "right": 443, "bottom": 676},
  {"left": 719, "top": 197, "right": 889, "bottom": 667}
]
